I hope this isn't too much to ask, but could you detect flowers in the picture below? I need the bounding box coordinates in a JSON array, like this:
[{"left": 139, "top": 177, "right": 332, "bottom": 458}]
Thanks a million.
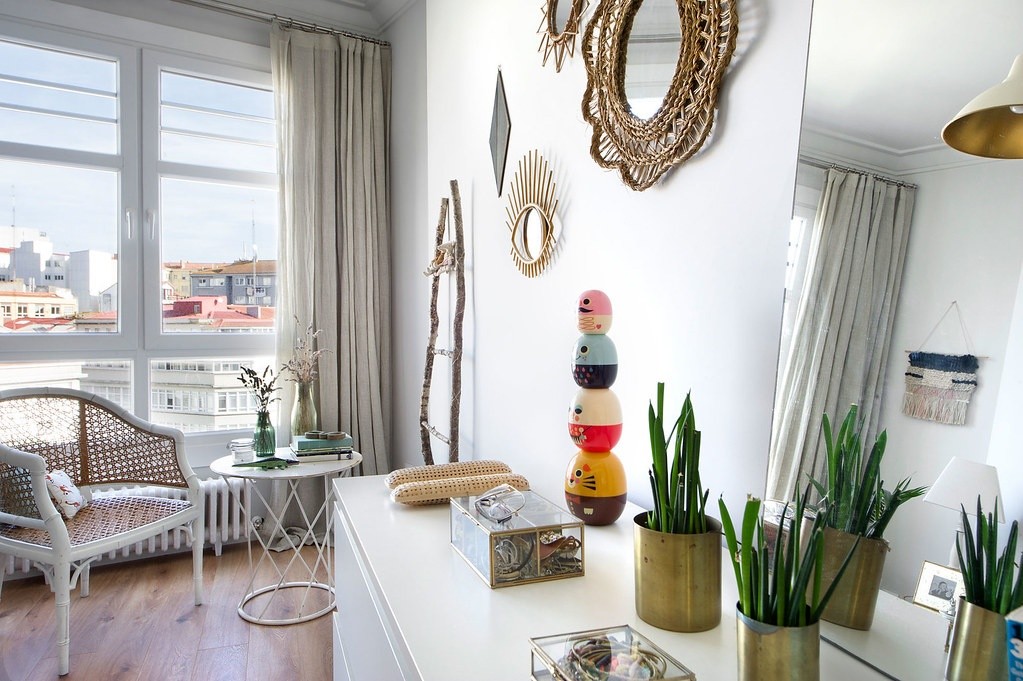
[{"left": 237, "top": 314, "right": 334, "bottom": 455}]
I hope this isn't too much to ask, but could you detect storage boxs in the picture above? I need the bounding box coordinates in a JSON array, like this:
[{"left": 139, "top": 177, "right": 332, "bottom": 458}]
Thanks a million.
[
  {"left": 449, "top": 490, "right": 586, "bottom": 590},
  {"left": 530, "top": 625, "right": 697, "bottom": 681}
]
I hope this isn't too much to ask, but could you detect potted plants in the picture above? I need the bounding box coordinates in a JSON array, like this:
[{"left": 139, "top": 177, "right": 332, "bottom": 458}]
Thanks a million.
[
  {"left": 943, "top": 493, "right": 1023, "bottom": 681},
  {"left": 805, "top": 402, "right": 930, "bottom": 631},
  {"left": 633, "top": 382, "right": 722, "bottom": 633},
  {"left": 718, "top": 493, "right": 864, "bottom": 681}
]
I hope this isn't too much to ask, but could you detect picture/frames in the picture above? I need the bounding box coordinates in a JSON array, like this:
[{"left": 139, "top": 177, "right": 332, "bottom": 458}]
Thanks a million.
[{"left": 912, "top": 559, "right": 967, "bottom": 615}]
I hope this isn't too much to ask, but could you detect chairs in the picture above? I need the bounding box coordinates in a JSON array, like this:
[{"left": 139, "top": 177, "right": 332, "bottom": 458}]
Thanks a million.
[{"left": 0, "top": 388, "right": 202, "bottom": 678}]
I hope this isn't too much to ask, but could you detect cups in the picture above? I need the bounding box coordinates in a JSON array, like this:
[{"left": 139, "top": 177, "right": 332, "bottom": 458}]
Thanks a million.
[{"left": 228, "top": 438, "right": 254, "bottom": 464}]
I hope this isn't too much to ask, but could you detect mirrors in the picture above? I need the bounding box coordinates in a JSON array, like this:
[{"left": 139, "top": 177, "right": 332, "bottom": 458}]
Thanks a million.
[
  {"left": 761, "top": 0, "right": 1023, "bottom": 681},
  {"left": 579, "top": 0, "right": 739, "bottom": 193},
  {"left": 538, "top": 0, "right": 591, "bottom": 72}
]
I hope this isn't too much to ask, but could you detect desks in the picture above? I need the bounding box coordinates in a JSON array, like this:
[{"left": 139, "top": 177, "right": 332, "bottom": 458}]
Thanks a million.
[{"left": 209, "top": 448, "right": 363, "bottom": 625}]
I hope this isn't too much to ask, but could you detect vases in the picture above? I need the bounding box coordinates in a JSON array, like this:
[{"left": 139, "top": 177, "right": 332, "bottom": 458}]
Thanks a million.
[
  {"left": 291, "top": 383, "right": 317, "bottom": 435},
  {"left": 254, "top": 412, "right": 275, "bottom": 457}
]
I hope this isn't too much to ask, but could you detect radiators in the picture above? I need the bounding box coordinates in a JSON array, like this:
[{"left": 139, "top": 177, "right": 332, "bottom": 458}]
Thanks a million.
[{"left": 1, "top": 476, "right": 265, "bottom": 576}]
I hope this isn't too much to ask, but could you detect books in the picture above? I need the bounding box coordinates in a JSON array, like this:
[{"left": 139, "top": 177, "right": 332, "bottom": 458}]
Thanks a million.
[{"left": 290, "top": 433, "right": 353, "bottom": 463}]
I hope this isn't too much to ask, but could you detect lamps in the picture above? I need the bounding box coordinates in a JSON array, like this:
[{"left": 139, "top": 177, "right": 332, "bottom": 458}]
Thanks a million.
[
  {"left": 924, "top": 459, "right": 1006, "bottom": 569},
  {"left": 941, "top": 51, "right": 1023, "bottom": 159}
]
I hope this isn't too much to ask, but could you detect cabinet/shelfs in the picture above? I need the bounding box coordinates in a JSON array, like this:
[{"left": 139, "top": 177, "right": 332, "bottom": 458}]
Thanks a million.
[{"left": 332, "top": 471, "right": 896, "bottom": 681}]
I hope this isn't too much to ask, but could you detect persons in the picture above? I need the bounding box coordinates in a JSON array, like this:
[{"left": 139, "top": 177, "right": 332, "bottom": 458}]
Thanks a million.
[
  {"left": 943, "top": 588, "right": 953, "bottom": 600},
  {"left": 930, "top": 582, "right": 947, "bottom": 599}
]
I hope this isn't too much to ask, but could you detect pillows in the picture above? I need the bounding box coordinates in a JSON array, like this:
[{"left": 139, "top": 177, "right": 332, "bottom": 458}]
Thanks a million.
[{"left": 0, "top": 466, "right": 87, "bottom": 527}]
